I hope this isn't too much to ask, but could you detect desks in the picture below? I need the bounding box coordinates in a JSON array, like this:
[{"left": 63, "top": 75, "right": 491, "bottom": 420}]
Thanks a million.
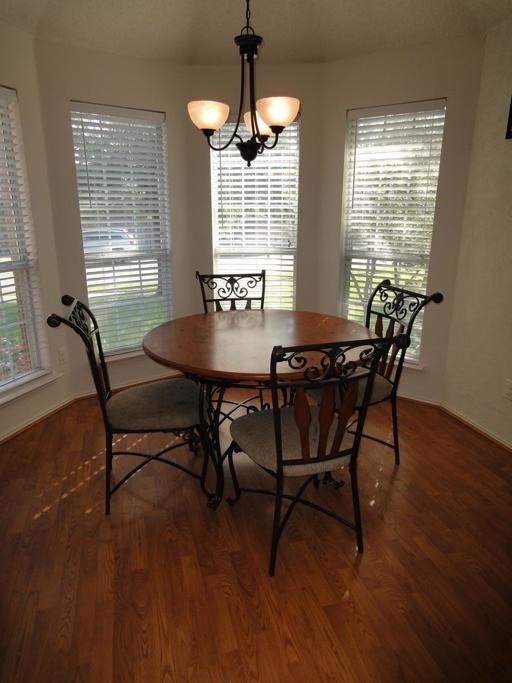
[{"left": 144, "top": 309, "right": 381, "bottom": 510}]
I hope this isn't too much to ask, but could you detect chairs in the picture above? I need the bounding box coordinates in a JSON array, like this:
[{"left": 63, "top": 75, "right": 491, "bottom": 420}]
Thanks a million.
[
  {"left": 190, "top": 271, "right": 267, "bottom": 452},
  {"left": 48, "top": 294, "right": 219, "bottom": 519},
  {"left": 216, "top": 335, "right": 409, "bottom": 577},
  {"left": 306, "top": 279, "right": 445, "bottom": 489}
]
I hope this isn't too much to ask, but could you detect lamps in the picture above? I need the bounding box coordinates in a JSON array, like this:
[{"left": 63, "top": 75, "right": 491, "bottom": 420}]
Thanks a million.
[{"left": 187, "top": 0, "right": 301, "bottom": 168}]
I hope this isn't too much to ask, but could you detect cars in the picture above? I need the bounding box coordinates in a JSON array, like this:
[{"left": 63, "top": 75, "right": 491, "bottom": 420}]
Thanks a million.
[{"left": 82, "top": 228, "right": 138, "bottom": 263}]
